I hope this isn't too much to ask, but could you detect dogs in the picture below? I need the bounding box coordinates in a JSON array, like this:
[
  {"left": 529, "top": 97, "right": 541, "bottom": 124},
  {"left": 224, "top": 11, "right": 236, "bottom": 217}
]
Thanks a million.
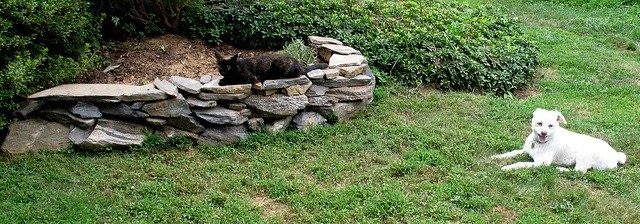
[{"left": 490, "top": 108, "right": 627, "bottom": 175}]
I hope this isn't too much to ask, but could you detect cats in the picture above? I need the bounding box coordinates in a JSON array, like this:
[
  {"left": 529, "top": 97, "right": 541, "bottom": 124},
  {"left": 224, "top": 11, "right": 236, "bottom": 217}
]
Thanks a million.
[{"left": 212, "top": 48, "right": 330, "bottom": 86}]
